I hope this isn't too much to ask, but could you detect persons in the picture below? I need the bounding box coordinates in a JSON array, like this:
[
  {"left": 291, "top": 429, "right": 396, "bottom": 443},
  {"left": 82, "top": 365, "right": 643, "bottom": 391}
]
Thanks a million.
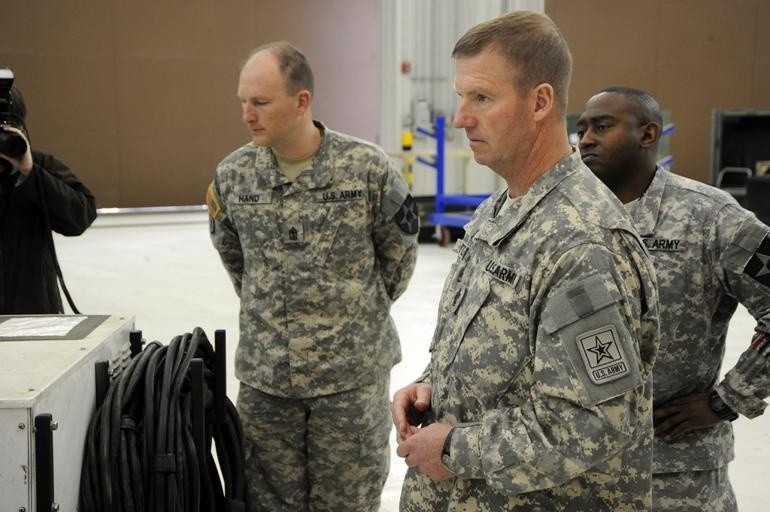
[
  {"left": 0, "top": 85, "right": 98, "bottom": 312},
  {"left": 567, "top": 86, "right": 769, "bottom": 512},
  {"left": 201, "top": 38, "right": 422, "bottom": 512},
  {"left": 387, "top": 9, "right": 655, "bottom": 512}
]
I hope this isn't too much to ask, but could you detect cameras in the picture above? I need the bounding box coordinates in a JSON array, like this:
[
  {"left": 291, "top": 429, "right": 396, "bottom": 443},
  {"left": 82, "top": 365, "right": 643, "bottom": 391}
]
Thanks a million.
[{"left": 0, "top": 67, "right": 27, "bottom": 177}]
